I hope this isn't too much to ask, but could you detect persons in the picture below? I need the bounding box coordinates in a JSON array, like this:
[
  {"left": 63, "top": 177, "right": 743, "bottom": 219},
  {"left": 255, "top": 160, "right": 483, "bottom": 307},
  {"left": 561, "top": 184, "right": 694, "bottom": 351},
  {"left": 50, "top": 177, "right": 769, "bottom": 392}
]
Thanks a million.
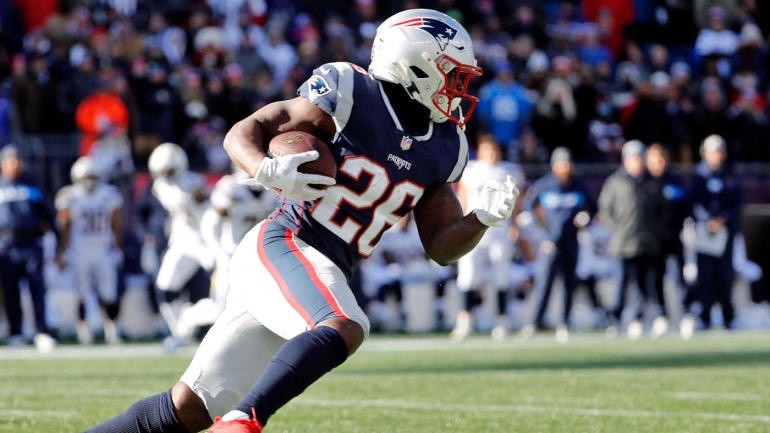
[
  {"left": 520, "top": 148, "right": 590, "bottom": 339},
  {"left": 140, "top": 144, "right": 213, "bottom": 348},
  {"left": 1, "top": 144, "right": 64, "bottom": 348},
  {"left": 687, "top": 136, "right": 743, "bottom": 329},
  {"left": 133, "top": 176, "right": 209, "bottom": 337},
  {"left": 54, "top": 156, "right": 126, "bottom": 345},
  {"left": 179, "top": 159, "right": 279, "bottom": 343},
  {"left": 453, "top": 135, "right": 524, "bottom": 342},
  {"left": 515, "top": 196, "right": 608, "bottom": 330},
  {"left": 643, "top": 145, "right": 694, "bottom": 335},
  {"left": 598, "top": 140, "right": 656, "bottom": 338},
  {"left": 376, "top": 210, "right": 451, "bottom": 332},
  {"left": 1, "top": 1, "right": 768, "bottom": 169},
  {"left": 83, "top": 9, "right": 519, "bottom": 433}
]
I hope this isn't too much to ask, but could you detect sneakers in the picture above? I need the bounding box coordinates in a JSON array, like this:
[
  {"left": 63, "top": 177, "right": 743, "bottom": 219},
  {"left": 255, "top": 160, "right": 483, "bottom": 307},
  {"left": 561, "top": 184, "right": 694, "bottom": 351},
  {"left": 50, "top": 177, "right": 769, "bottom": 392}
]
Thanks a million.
[{"left": 205, "top": 417, "right": 263, "bottom": 433}]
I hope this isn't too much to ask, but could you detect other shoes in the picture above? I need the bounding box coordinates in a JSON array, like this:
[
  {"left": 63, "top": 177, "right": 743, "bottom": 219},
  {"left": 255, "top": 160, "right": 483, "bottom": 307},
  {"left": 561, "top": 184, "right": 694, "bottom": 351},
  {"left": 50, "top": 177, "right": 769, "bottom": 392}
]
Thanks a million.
[
  {"left": 4, "top": 335, "right": 24, "bottom": 346},
  {"left": 162, "top": 333, "right": 190, "bottom": 349},
  {"left": 104, "top": 320, "right": 119, "bottom": 343},
  {"left": 75, "top": 322, "right": 92, "bottom": 344},
  {"left": 33, "top": 333, "right": 54, "bottom": 351}
]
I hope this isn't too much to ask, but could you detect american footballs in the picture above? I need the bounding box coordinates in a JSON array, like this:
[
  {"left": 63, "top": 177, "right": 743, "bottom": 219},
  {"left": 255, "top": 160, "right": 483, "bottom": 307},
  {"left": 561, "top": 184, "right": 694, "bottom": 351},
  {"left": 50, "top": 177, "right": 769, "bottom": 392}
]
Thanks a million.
[{"left": 266, "top": 131, "right": 337, "bottom": 193}]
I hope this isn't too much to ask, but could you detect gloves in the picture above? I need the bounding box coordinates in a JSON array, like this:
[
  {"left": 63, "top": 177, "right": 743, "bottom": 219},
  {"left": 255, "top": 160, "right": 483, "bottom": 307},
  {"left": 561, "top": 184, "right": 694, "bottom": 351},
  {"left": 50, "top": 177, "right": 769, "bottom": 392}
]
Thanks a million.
[
  {"left": 253, "top": 150, "right": 336, "bottom": 201},
  {"left": 475, "top": 175, "right": 520, "bottom": 226}
]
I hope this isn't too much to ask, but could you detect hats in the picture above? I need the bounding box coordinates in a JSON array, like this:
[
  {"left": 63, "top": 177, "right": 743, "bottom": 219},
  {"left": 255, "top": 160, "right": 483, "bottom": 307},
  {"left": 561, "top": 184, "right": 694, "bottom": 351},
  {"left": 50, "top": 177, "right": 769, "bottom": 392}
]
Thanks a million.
[
  {"left": 548, "top": 146, "right": 572, "bottom": 166},
  {"left": 621, "top": 141, "right": 648, "bottom": 158},
  {"left": 704, "top": 135, "right": 726, "bottom": 152}
]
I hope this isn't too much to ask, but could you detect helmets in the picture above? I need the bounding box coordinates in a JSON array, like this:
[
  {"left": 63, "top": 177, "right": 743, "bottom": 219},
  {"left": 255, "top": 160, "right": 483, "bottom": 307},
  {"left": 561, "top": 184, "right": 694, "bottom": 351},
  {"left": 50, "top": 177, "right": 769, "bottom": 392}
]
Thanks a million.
[
  {"left": 148, "top": 142, "right": 187, "bottom": 179},
  {"left": 365, "top": 7, "right": 475, "bottom": 123},
  {"left": 70, "top": 156, "right": 100, "bottom": 185}
]
